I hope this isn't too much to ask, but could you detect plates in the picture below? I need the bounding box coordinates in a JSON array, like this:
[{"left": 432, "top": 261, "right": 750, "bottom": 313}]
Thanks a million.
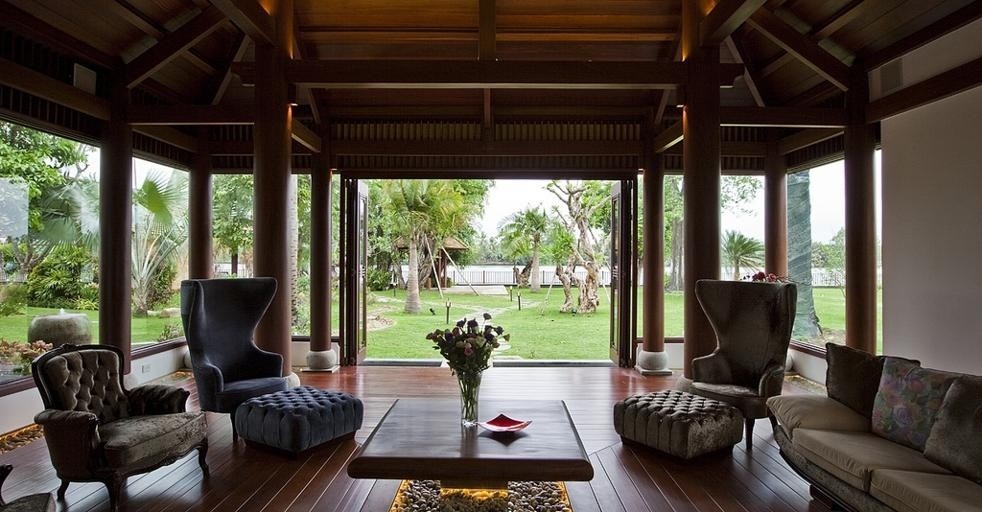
[{"left": 476, "top": 414, "right": 532, "bottom": 433}]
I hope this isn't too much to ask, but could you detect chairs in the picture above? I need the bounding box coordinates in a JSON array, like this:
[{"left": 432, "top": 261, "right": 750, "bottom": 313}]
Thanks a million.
[{"left": 33, "top": 344, "right": 211, "bottom": 512}]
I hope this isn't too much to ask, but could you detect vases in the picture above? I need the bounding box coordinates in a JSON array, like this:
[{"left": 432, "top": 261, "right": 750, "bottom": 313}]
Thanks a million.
[{"left": 461, "top": 372, "right": 482, "bottom": 433}]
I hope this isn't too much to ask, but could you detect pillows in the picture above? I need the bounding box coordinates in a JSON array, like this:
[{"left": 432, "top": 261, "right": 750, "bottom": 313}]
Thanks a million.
[
  {"left": 871, "top": 357, "right": 957, "bottom": 451},
  {"left": 925, "top": 383, "right": 982, "bottom": 485},
  {"left": 822, "top": 342, "right": 920, "bottom": 415}
]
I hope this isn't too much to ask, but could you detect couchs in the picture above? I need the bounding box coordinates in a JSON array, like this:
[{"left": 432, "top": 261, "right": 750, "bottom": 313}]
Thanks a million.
[
  {"left": 766, "top": 342, "right": 982, "bottom": 512},
  {"left": 685, "top": 282, "right": 798, "bottom": 450},
  {"left": 234, "top": 384, "right": 364, "bottom": 458},
  {"left": 182, "top": 279, "right": 299, "bottom": 442},
  {"left": 613, "top": 388, "right": 744, "bottom": 458}
]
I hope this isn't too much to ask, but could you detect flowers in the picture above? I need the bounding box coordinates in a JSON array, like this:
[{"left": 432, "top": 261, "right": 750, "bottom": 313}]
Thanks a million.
[{"left": 429, "top": 311, "right": 509, "bottom": 413}]
